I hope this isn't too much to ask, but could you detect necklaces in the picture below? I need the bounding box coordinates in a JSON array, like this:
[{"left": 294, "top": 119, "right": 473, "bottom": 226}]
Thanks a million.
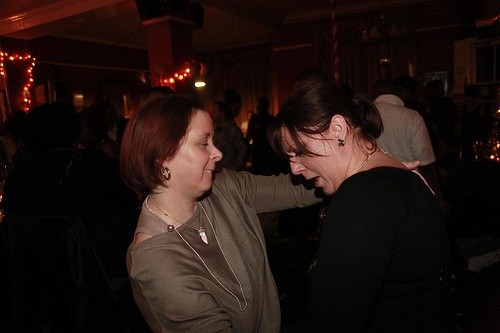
[
  {"left": 146, "top": 191, "right": 248, "bottom": 312},
  {"left": 354, "top": 147, "right": 381, "bottom": 173},
  {"left": 150, "top": 195, "right": 210, "bottom": 246}
]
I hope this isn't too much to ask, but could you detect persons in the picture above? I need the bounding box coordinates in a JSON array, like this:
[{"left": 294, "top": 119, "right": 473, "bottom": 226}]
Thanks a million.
[
  {"left": 121, "top": 95, "right": 436, "bottom": 333},
  {"left": 267, "top": 80, "right": 472, "bottom": 332},
  {"left": 222, "top": 92, "right": 246, "bottom": 130},
  {"left": 0, "top": 95, "right": 120, "bottom": 332},
  {"left": 369, "top": 78, "right": 445, "bottom": 214},
  {"left": 209, "top": 102, "right": 246, "bottom": 172},
  {"left": 469, "top": 250, "right": 499, "bottom": 274},
  {"left": 246, "top": 95, "right": 290, "bottom": 176},
  {"left": 142, "top": 86, "right": 176, "bottom": 106}
]
